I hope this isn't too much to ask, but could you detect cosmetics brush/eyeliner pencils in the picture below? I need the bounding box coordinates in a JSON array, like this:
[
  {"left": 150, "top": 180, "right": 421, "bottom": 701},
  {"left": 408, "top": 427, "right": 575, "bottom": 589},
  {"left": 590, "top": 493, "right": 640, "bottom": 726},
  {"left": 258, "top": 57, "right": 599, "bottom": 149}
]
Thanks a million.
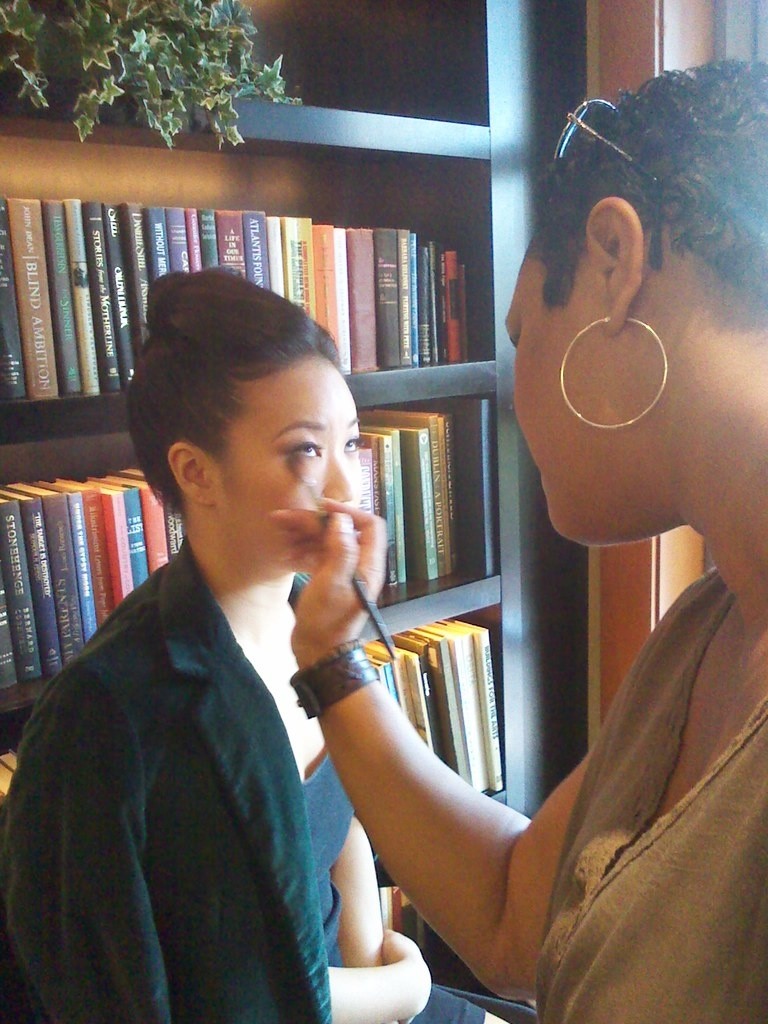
[{"left": 287, "top": 453, "right": 398, "bottom": 664}]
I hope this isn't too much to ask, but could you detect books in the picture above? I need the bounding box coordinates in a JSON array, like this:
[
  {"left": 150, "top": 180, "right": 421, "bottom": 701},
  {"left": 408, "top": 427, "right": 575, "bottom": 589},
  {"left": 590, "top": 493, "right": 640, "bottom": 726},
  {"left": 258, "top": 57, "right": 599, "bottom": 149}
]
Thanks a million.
[{"left": 0, "top": 198, "right": 505, "bottom": 952}]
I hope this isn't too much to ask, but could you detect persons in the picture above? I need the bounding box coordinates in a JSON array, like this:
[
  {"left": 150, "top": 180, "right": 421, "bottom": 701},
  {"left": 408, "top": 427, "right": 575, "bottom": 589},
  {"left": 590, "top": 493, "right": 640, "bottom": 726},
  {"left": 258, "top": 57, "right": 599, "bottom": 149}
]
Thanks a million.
[
  {"left": 0, "top": 267, "right": 513, "bottom": 1024},
  {"left": 294, "top": 58, "right": 767, "bottom": 1024}
]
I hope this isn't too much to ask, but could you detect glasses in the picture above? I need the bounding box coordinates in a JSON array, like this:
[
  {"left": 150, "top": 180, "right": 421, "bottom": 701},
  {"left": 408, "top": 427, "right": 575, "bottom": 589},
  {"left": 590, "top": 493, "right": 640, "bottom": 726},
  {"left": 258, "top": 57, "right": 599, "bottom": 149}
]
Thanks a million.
[{"left": 554, "top": 100, "right": 668, "bottom": 270}]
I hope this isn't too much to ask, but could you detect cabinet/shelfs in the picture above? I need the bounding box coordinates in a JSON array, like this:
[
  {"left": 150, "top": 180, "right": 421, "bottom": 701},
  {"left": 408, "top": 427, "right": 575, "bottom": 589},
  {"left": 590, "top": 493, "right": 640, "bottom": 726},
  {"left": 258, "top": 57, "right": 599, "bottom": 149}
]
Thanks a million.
[{"left": 0, "top": 0, "right": 768, "bottom": 1024}]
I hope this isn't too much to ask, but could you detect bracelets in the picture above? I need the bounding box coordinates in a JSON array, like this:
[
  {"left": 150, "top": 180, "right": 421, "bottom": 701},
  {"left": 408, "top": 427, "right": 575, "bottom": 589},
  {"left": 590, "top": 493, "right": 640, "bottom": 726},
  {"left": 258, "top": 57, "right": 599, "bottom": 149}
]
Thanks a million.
[{"left": 289, "top": 649, "right": 381, "bottom": 719}]
